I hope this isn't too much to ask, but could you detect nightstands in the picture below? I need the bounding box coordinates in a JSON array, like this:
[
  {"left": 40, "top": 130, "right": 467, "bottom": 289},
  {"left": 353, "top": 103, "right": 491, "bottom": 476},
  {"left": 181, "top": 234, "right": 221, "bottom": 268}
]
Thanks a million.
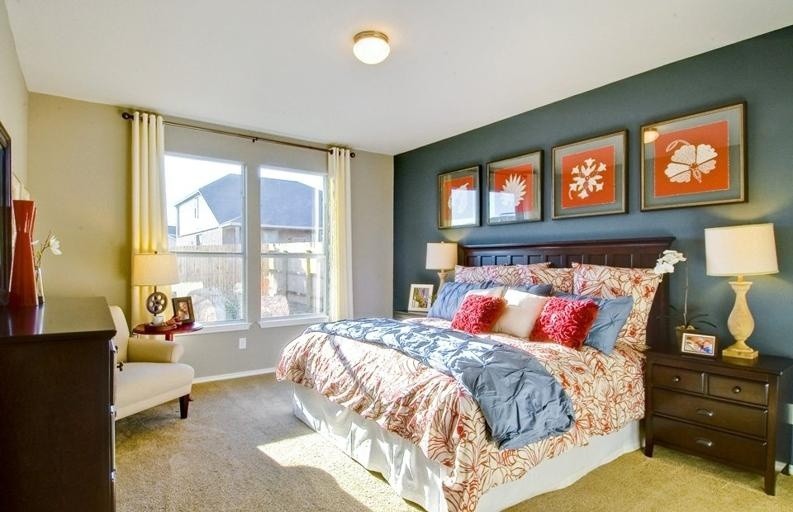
[
  {"left": 643, "top": 343, "right": 793, "bottom": 496},
  {"left": 393, "top": 310, "right": 429, "bottom": 322}
]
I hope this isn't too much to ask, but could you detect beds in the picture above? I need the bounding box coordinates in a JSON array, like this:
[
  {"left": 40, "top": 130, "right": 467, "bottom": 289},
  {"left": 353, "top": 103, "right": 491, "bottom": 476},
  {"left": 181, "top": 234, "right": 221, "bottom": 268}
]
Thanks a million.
[{"left": 291, "top": 236, "right": 679, "bottom": 512}]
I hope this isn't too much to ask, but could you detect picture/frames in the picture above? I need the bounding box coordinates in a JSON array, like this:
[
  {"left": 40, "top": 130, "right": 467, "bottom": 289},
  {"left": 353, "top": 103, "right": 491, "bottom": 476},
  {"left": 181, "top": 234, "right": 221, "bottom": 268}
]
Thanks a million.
[
  {"left": 408, "top": 283, "right": 433, "bottom": 312},
  {"left": 640, "top": 100, "right": 748, "bottom": 213},
  {"left": 437, "top": 164, "right": 484, "bottom": 230},
  {"left": 486, "top": 150, "right": 545, "bottom": 226},
  {"left": 551, "top": 128, "right": 630, "bottom": 221},
  {"left": 172, "top": 296, "right": 195, "bottom": 323},
  {"left": 681, "top": 333, "right": 716, "bottom": 357}
]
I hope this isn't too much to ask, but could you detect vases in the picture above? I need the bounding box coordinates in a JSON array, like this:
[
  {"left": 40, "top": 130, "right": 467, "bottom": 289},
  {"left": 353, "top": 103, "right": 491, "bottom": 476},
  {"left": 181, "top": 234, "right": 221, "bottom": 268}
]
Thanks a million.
[
  {"left": 675, "top": 325, "right": 698, "bottom": 354},
  {"left": 8, "top": 200, "right": 38, "bottom": 307},
  {"left": 34, "top": 266, "right": 46, "bottom": 306}
]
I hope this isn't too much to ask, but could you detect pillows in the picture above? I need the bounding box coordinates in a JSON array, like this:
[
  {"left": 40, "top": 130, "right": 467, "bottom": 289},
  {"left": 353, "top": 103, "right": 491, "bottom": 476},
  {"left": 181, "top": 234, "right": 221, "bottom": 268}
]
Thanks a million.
[{"left": 427, "top": 261, "right": 664, "bottom": 357}]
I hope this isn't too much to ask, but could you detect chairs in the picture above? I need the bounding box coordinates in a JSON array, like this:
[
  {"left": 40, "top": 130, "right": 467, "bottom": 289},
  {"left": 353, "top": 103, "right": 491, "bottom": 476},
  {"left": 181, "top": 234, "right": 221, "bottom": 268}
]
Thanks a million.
[{"left": 108, "top": 305, "right": 195, "bottom": 422}]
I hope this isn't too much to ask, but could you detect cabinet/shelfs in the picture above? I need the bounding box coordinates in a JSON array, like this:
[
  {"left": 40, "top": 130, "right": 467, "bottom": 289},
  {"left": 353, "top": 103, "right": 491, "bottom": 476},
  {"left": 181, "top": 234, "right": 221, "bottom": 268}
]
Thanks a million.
[{"left": 0, "top": 296, "right": 118, "bottom": 512}]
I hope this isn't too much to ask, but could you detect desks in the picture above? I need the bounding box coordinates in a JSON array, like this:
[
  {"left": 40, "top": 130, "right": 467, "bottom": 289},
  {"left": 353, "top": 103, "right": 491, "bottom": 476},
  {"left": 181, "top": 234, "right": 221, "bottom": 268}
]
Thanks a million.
[{"left": 132, "top": 323, "right": 203, "bottom": 401}]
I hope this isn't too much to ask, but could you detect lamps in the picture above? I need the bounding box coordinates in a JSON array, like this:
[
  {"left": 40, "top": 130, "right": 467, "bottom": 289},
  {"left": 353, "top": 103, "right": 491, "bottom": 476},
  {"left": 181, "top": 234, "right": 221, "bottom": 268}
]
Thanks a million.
[
  {"left": 425, "top": 240, "right": 459, "bottom": 296},
  {"left": 352, "top": 31, "right": 391, "bottom": 65},
  {"left": 703, "top": 222, "right": 780, "bottom": 359},
  {"left": 133, "top": 251, "right": 181, "bottom": 324}
]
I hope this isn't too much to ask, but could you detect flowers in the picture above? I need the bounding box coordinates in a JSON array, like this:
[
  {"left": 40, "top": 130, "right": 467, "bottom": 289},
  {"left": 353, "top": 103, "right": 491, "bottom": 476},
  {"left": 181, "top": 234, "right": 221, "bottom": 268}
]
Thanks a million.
[
  {"left": 30, "top": 228, "right": 63, "bottom": 282},
  {"left": 646, "top": 248, "right": 720, "bottom": 329}
]
